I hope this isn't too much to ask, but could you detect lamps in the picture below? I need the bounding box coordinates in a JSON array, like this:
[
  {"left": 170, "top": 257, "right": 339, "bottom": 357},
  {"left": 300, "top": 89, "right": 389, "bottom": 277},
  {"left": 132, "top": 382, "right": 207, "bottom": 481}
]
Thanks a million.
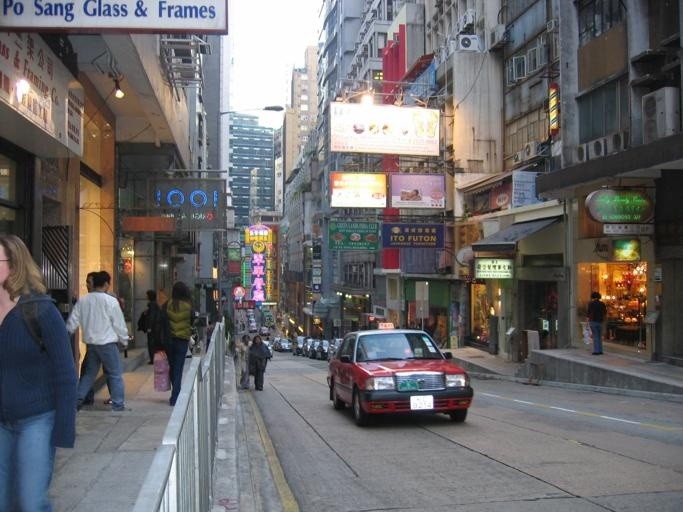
[{"left": 113, "top": 79, "right": 124, "bottom": 98}]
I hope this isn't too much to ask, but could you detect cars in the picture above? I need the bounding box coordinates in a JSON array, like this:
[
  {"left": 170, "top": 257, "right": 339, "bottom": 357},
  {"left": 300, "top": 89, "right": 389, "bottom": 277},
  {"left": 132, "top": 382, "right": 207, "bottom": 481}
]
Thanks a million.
[{"left": 245, "top": 306, "right": 473, "bottom": 426}]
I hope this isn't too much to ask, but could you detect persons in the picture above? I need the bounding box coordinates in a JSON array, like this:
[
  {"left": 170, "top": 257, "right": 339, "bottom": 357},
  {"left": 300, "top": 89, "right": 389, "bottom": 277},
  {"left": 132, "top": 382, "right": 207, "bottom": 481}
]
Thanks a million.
[
  {"left": 237, "top": 333, "right": 250, "bottom": 389},
  {"left": 586, "top": 292, "right": 607, "bottom": 355},
  {"left": 142, "top": 289, "right": 159, "bottom": 366},
  {"left": 400, "top": 189, "right": 421, "bottom": 201},
  {"left": 64, "top": 270, "right": 128, "bottom": 412},
  {"left": 0, "top": 233, "right": 76, "bottom": 512},
  {"left": 246, "top": 334, "right": 272, "bottom": 391},
  {"left": 151, "top": 280, "right": 195, "bottom": 405},
  {"left": 77, "top": 271, "right": 122, "bottom": 405}
]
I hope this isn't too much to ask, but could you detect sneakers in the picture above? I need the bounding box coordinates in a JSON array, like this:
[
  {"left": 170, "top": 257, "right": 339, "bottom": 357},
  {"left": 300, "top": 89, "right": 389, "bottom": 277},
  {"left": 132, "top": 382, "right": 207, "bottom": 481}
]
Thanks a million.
[
  {"left": 112, "top": 405, "right": 132, "bottom": 411},
  {"left": 592, "top": 352, "right": 603, "bottom": 355},
  {"left": 103, "top": 398, "right": 113, "bottom": 404}
]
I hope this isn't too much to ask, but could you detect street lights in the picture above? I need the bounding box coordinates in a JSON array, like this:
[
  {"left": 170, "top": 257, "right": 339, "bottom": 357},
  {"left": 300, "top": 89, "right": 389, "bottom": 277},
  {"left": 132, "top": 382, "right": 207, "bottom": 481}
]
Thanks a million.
[{"left": 217, "top": 105, "right": 284, "bottom": 313}]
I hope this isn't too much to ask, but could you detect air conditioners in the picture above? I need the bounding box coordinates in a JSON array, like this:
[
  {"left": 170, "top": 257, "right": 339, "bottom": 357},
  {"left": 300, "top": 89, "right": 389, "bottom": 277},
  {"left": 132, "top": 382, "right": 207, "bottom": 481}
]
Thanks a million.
[
  {"left": 547, "top": 19, "right": 559, "bottom": 33},
  {"left": 571, "top": 144, "right": 589, "bottom": 164},
  {"left": 607, "top": 129, "right": 630, "bottom": 156},
  {"left": 487, "top": 24, "right": 506, "bottom": 51},
  {"left": 524, "top": 141, "right": 541, "bottom": 160},
  {"left": 434, "top": 8, "right": 479, "bottom": 71},
  {"left": 642, "top": 87, "right": 681, "bottom": 144},
  {"left": 513, "top": 151, "right": 524, "bottom": 168},
  {"left": 588, "top": 137, "right": 606, "bottom": 160}
]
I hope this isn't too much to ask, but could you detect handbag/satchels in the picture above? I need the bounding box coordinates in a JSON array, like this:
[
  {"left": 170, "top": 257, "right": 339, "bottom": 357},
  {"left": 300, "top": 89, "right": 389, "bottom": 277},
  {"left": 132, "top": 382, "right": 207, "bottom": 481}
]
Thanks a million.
[{"left": 153, "top": 351, "right": 171, "bottom": 392}]
